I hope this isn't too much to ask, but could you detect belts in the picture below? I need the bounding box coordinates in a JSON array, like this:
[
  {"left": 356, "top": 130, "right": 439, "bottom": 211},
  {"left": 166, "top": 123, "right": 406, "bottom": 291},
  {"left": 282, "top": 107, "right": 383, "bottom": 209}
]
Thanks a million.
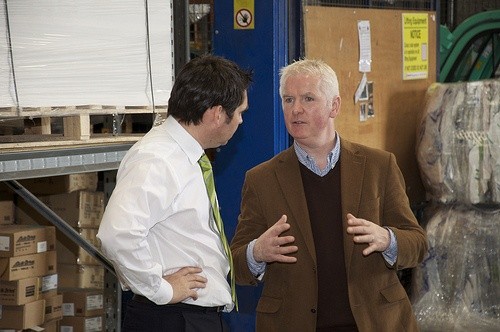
[{"left": 122, "top": 289, "right": 225, "bottom": 313}]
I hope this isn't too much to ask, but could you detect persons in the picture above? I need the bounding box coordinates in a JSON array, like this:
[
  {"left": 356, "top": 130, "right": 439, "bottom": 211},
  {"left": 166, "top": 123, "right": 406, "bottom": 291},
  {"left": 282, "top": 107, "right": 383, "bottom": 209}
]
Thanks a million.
[
  {"left": 95, "top": 53, "right": 254, "bottom": 332},
  {"left": 229, "top": 56, "right": 428, "bottom": 332}
]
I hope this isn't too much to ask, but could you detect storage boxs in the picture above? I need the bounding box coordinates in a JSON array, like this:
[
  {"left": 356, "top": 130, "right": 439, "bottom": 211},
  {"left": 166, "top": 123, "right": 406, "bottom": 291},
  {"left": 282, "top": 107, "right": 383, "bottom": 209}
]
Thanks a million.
[{"left": 0, "top": 172, "right": 106, "bottom": 332}]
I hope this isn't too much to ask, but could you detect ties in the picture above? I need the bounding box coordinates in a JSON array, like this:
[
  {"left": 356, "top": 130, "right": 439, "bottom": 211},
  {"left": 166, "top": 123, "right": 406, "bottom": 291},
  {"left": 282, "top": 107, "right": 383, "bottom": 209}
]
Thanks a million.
[{"left": 198, "top": 155, "right": 239, "bottom": 312}]
